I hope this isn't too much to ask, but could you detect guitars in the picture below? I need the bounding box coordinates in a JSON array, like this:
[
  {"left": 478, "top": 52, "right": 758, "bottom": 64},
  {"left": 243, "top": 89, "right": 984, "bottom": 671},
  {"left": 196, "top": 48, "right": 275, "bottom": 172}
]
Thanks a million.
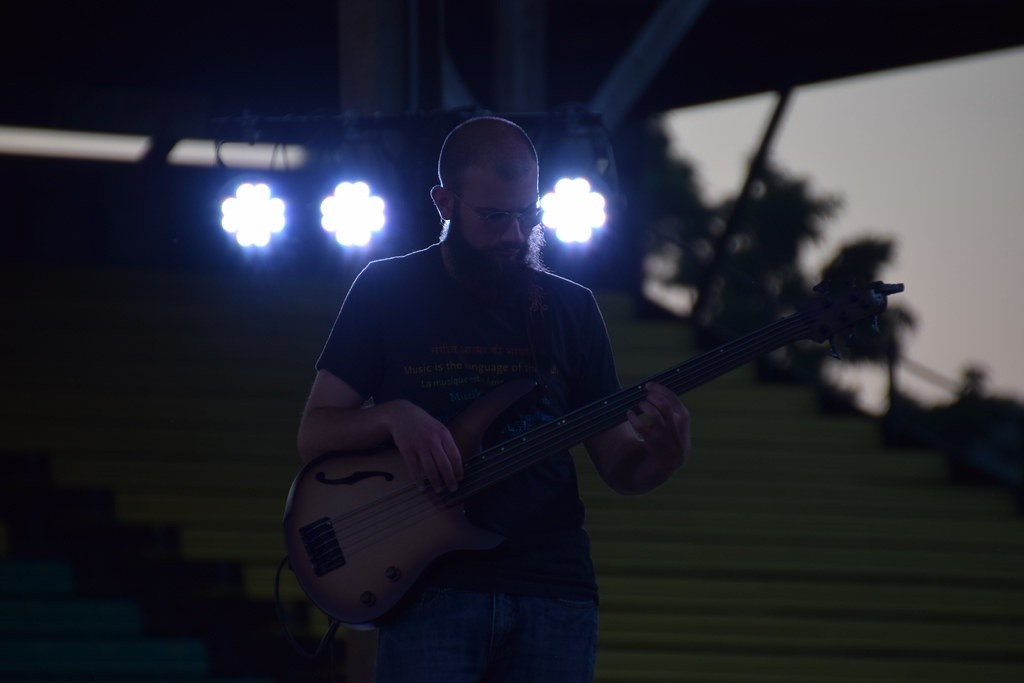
[{"left": 283, "top": 279, "right": 908, "bottom": 633}]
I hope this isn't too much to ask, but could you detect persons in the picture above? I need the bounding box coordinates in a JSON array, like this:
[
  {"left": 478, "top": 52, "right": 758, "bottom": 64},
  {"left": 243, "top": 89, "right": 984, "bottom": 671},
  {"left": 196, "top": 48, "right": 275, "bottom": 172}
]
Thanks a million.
[{"left": 277, "top": 115, "right": 695, "bottom": 683}]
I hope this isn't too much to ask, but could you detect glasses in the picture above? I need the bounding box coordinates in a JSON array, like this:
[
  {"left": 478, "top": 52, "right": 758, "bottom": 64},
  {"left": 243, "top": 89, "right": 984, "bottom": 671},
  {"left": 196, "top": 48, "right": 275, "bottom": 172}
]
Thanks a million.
[{"left": 449, "top": 188, "right": 544, "bottom": 227}]
